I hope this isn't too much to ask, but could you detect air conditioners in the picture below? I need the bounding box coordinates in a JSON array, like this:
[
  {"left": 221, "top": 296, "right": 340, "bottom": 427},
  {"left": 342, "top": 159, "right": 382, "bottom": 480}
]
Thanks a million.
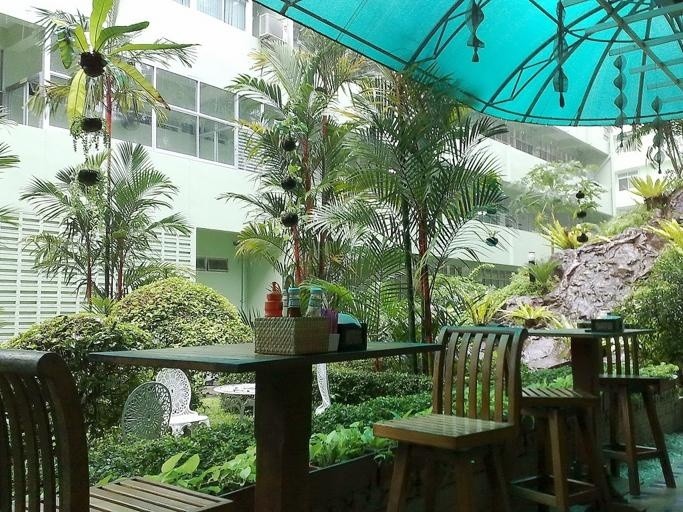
[{"left": 258, "top": 12, "right": 289, "bottom": 45}]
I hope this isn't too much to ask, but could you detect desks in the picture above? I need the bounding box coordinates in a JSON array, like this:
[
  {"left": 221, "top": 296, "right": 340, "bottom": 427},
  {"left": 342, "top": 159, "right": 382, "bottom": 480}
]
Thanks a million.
[
  {"left": 528, "top": 329, "right": 656, "bottom": 511},
  {"left": 212, "top": 383, "right": 255, "bottom": 424}
]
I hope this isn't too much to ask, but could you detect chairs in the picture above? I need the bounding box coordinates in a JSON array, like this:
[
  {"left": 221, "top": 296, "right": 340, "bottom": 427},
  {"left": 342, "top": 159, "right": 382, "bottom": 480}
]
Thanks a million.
[
  {"left": 0, "top": 348, "right": 237, "bottom": 510},
  {"left": 504, "top": 336, "right": 611, "bottom": 511},
  {"left": 593, "top": 334, "right": 677, "bottom": 496},
  {"left": 372, "top": 327, "right": 527, "bottom": 511}
]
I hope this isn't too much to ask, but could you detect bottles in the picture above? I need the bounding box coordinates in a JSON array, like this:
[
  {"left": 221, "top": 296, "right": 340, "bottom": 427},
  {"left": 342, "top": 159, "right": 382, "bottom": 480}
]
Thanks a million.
[
  {"left": 304, "top": 287, "right": 323, "bottom": 318},
  {"left": 264, "top": 281, "right": 283, "bottom": 317},
  {"left": 286, "top": 287, "right": 302, "bottom": 318}
]
